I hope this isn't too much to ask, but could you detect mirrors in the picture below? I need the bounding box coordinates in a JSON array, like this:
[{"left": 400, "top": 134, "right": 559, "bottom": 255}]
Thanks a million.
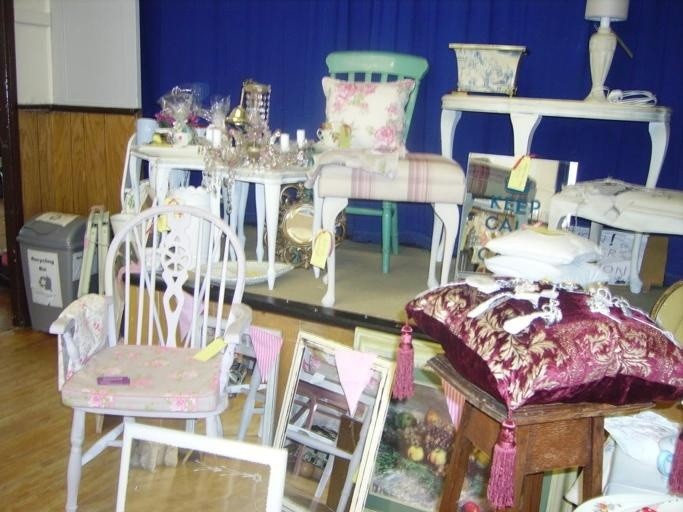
[{"left": 115, "top": 421, "right": 289, "bottom": 508}]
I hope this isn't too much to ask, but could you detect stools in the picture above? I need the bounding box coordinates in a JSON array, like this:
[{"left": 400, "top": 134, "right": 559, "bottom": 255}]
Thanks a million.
[
  {"left": 313, "top": 153, "right": 466, "bottom": 308},
  {"left": 554, "top": 176, "right": 682, "bottom": 235}
]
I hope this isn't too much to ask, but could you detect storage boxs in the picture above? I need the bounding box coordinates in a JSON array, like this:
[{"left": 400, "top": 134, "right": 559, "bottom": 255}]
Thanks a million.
[{"left": 444, "top": 41, "right": 528, "bottom": 97}]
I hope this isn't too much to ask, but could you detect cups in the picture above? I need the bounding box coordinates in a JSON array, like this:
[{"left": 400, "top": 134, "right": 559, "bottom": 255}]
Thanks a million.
[{"left": 136, "top": 119, "right": 157, "bottom": 146}]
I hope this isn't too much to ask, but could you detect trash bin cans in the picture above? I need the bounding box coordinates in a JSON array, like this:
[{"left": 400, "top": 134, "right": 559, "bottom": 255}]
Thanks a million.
[{"left": 16, "top": 212, "right": 98, "bottom": 336}]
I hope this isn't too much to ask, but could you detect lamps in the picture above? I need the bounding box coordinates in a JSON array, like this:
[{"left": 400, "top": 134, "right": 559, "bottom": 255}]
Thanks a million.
[{"left": 581, "top": 0, "right": 634, "bottom": 99}]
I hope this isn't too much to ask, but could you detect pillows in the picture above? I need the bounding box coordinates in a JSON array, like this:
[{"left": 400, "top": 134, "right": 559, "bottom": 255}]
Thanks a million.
[
  {"left": 563, "top": 408, "right": 682, "bottom": 505},
  {"left": 321, "top": 76, "right": 412, "bottom": 156},
  {"left": 406, "top": 274, "right": 682, "bottom": 411},
  {"left": 483, "top": 226, "right": 608, "bottom": 289}
]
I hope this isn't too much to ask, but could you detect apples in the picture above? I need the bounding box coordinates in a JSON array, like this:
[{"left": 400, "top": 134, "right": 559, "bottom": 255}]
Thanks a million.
[
  {"left": 430, "top": 447, "right": 448, "bottom": 465},
  {"left": 425, "top": 409, "right": 438, "bottom": 424},
  {"left": 407, "top": 445, "right": 424, "bottom": 462},
  {"left": 398, "top": 412, "right": 417, "bottom": 429}
]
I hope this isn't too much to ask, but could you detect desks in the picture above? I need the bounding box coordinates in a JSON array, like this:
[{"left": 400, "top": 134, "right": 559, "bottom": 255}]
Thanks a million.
[
  {"left": 426, "top": 350, "right": 656, "bottom": 506},
  {"left": 441, "top": 91, "right": 680, "bottom": 286},
  {"left": 130, "top": 125, "right": 309, "bottom": 288}
]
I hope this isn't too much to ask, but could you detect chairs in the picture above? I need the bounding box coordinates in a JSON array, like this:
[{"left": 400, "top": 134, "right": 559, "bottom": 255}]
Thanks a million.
[
  {"left": 50, "top": 204, "right": 251, "bottom": 507},
  {"left": 324, "top": 43, "right": 429, "bottom": 274},
  {"left": 651, "top": 279, "right": 682, "bottom": 410}
]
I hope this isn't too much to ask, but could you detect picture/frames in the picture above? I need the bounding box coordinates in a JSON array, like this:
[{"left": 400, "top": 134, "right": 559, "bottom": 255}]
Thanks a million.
[
  {"left": 348, "top": 326, "right": 578, "bottom": 511},
  {"left": 271, "top": 329, "right": 396, "bottom": 509}
]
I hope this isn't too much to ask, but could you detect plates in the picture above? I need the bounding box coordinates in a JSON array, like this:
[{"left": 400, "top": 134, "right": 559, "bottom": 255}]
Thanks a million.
[{"left": 573, "top": 493, "right": 683, "bottom": 511}]
